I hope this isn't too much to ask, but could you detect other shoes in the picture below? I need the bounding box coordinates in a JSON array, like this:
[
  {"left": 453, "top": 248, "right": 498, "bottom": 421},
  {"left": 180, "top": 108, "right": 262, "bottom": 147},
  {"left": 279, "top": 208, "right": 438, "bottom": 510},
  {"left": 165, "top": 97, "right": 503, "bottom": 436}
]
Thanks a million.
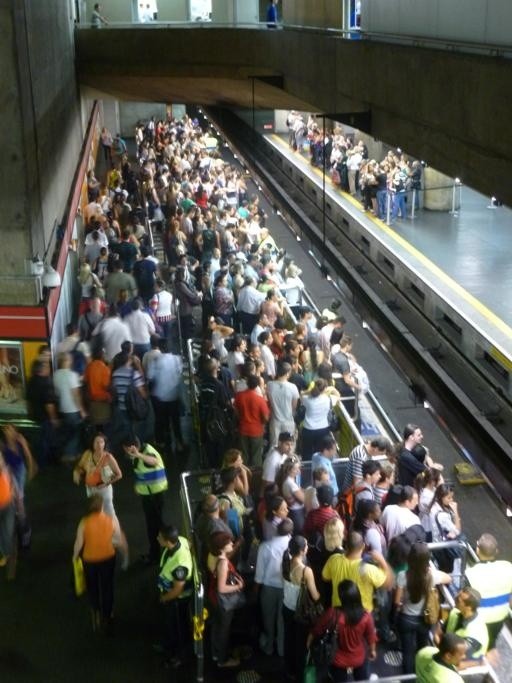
[
  {"left": 384, "top": 631, "right": 397, "bottom": 641},
  {"left": 213, "top": 655, "right": 242, "bottom": 668}
]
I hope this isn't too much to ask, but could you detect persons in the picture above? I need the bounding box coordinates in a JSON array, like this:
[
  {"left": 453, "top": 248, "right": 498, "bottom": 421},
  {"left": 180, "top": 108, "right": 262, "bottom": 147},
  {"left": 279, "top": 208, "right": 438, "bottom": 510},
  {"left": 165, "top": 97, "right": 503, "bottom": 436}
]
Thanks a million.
[
  {"left": 54, "top": 107, "right": 369, "bottom": 415},
  {"left": 369, "top": 147, "right": 426, "bottom": 224},
  {"left": 145, "top": 3, "right": 153, "bottom": 21},
  {"left": 24, "top": 357, "right": 62, "bottom": 475},
  {"left": 265, "top": 0, "right": 280, "bottom": 29},
  {"left": 91, "top": 3, "right": 112, "bottom": 29},
  {"left": 2, "top": 415, "right": 512, "bottom": 683},
  {"left": 139, "top": 3, "right": 145, "bottom": 22},
  {"left": 37, "top": 345, "right": 53, "bottom": 361}
]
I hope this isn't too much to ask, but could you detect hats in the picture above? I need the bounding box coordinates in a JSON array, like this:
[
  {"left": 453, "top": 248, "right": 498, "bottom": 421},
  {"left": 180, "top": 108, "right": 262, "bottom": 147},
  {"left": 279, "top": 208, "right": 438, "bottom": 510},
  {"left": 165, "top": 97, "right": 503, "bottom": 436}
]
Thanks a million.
[
  {"left": 298, "top": 306, "right": 316, "bottom": 313},
  {"left": 313, "top": 361, "right": 333, "bottom": 385}
]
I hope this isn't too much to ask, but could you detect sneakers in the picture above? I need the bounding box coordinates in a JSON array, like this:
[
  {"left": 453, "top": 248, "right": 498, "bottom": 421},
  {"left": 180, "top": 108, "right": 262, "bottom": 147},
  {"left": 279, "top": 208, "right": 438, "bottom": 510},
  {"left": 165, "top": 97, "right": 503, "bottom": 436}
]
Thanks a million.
[
  {"left": 161, "top": 654, "right": 194, "bottom": 669},
  {"left": 149, "top": 439, "right": 169, "bottom": 449},
  {"left": 176, "top": 441, "right": 190, "bottom": 452},
  {"left": 0, "top": 528, "right": 31, "bottom": 582},
  {"left": 151, "top": 639, "right": 179, "bottom": 653}
]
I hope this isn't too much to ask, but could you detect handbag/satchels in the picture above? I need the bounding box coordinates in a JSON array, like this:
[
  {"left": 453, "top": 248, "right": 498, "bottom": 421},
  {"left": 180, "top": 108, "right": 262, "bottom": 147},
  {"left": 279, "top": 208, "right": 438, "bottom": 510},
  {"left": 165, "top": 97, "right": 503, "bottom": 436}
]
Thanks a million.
[
  {"left": 215, "top": 493, "right": 242, "bottom": 541},
  {"left": 436, "top": 509, "right": 460, "bottom": 573},
  {"left": 69, "top": 162, "right": 166, "bottom": 419},
  {"left": 295, "top": 567, "right": 325, "bottom": 628},
  {"left": 311, "top": 606, "right": 341, "bottom": 670},
  {"left": 110, "top": 514, "right": 125, "bottom": 557},
  {"left": 80, "top": 416, "right": 105, "bottom": 451},
  {"left": 73, "top": 557, "right": 89, "bottom": 598},
  {"left": 423, "top": 569, "right": 441, "bottom": 626},
  {"left": 214, "top": 558, "right": 247, "bottom": 612},
  {"left": 100, "top": 452, "right": 117, "bottom": 485}
]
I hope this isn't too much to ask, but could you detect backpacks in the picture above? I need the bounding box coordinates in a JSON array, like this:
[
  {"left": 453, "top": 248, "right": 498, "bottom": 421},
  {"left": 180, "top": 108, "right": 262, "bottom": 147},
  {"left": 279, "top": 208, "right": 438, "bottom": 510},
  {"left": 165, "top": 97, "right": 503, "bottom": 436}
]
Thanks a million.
[
  {"left": 206, "top": 404, "right": 233, "bottom": 445},
  {"left": 48, "top": 424, "right": 74, "bottom": 460},
  {"left": 333, "top": 483, "right": 371, "bottom": 540},
  {"left": 202, "top": 229, "right": 217, "bottom": 254},
  {"left": 327, "top": 395, "right": 340, "bottom": 431},
  {"left": 293, "top": 398, "right": 305, "bottom": 424},
  {"left": 348, "top": 361, "right": 369, "bottom": 396}
]
